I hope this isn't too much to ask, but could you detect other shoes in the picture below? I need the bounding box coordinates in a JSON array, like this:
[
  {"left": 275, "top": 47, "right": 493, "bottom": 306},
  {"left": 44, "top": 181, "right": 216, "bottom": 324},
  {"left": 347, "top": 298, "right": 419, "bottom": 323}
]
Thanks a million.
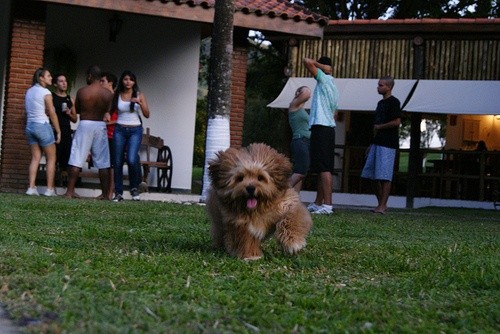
[
  {"left": 131, "top": 190, "right": 140, "bottom": 201},
  {"left": 44, "top": 187, "right": 58, "bottom": 196},
  {"left": 25, "top": 186, "right": 39, "bottom": 196},
  {"left": 112, "top": 192, "right": 124, "bottom": 202},
  {"left": 311, "top": 205, "right": 334, "bottom": 214},
  {"left": 306, "top": 203, "right": 323, "bottom": 213}
]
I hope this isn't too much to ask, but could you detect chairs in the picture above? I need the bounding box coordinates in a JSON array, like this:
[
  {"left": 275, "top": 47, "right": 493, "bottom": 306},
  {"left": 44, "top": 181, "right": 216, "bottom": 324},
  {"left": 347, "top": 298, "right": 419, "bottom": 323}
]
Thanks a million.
[{"left": 342, "top": 146, "right": 384, "bottom": 197}]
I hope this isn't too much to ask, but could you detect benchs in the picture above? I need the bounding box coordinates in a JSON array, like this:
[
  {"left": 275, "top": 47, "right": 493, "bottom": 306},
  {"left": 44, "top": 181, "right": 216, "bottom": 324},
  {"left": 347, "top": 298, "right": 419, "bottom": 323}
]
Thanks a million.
[{"left": 59, "top": 161, "right": 167, "bottom": 191}]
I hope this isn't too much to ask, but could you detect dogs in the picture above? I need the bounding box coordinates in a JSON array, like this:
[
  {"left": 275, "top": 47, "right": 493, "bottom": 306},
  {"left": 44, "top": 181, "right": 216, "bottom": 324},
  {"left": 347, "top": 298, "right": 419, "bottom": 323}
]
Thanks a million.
[{"left": 206, "top": 142, "right": 313, "bottom": 260}]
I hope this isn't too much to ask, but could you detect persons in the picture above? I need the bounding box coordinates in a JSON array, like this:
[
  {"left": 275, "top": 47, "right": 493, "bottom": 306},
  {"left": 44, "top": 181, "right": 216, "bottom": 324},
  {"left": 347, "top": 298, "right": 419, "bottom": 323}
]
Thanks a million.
[
  {"left": 24, "top": 68, "right": 61, "bottom": 197},
  {"left": 62, "top": 65, "right": 112, "bottom": 200},
  {"left": 47, "top": 71, "right": 77, "bottom": 187},
  {"left": 302, "top": 55, "right": 339, "bottom": 214},
  {"left": 112, "top": 70, "right": 150, "bottom": 202},
  {"left": 98, "top": 71, "right": 119, "bottom": 199},
  {"left": 289, "top": 86, "right": 311, "bottom": 196},
  {"left": 360, "top": 76, "right": 402, "bottom": 214}
]
